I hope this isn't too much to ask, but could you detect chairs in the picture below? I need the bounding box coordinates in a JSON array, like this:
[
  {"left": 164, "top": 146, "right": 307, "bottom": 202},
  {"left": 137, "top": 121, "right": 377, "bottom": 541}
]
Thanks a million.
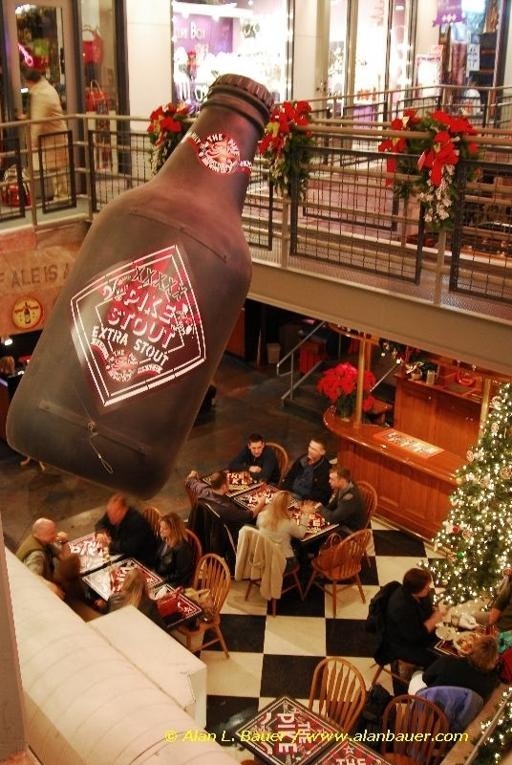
[
  {"left": 182, "top": 552, "right": 237, "bottom": 663},
  {"left": 303, "top": 529, "right": 372, "bottom": 617},
  {"left": 381, "top": 694, "right": 452, "bottom": 765},
  {"left": 262, "top": 443, "right": 289, "bottom": 487},
  {"left": 172, "top": 531, "right": 204, "bottom": 590},
  {"left": 196, "top": 499, "right": 236, "bottom": 558},
  {"left": 308, "top": 656, "right": 363, "bottom": 733},
  {"left": 369, "top": 580, "right": 424, "bottom": 690},
  {"left": 240, "top": 527, "right": 304, "bottom": 615},
  {"left": 354, "top": 480, "right": 378, "bottom": 530},
  {"left": 137, "top": 506, "right": 163, "bottom": 545}
]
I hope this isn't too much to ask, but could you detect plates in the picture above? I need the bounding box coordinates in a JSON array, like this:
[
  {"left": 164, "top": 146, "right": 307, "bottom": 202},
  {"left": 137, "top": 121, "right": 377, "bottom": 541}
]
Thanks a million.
[
  {"left": 452, "top": 631, "right": 482, "bottom": 655},
  {"left": 434, "top": 625, "right": 457, "bottom": 641}
]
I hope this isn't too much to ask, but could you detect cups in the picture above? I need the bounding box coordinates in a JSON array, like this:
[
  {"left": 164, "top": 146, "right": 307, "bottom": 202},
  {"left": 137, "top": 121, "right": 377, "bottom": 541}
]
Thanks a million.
[{"left": 450, "top": 605, "right": 462, "bottom": 629}]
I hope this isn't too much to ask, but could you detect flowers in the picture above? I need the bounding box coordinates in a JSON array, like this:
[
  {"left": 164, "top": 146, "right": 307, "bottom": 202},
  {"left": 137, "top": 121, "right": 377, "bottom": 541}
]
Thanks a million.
[
  {"left": 319, "top": 364, "right": 375, "bottom": 419},
  {"left": 383, "top": 110, "right": 479, "bottom": 226},
  {"left": 145, "top": 101, "right": 199, "bottom": 159},
  {"left": 263, "top": 99, "right": 311, "bottom": 205}
]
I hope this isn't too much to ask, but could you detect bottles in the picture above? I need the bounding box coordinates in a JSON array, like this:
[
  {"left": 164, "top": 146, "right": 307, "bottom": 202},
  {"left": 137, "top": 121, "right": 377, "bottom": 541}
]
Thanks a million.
[{"left": 6, "top": 72, "right": 275, "bottom": 500}]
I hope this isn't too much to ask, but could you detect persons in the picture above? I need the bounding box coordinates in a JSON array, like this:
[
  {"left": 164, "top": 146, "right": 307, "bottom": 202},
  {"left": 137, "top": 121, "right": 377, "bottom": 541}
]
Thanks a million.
[
  {"left": 292, "top": 468, "right": 367, "bottom": 577},
  {"left": 460, "top": 76, "right": 490, "bottom": 124},
  {"left": 0, "top": 356, "right": 15, "bottom": 387},
  {"left": 47, "top": 555, "right": 108, "bottom": 612},
  {"left": 106, "top": 571, "right": 166, "bottom": 630},
  {"left": 282, "top": 439, "right": 335, "bottom": 506},
  {"left": 408, "top": 637, "right": 499, "bottom": 699},
  {"left": 229, "top": 435, "right": 276, "bottom": 483},
  {"left": 21, "top": 71, "right": 70, "bottom": 196},
  {"left": 486, "top": 577, "right": 512, "bottom": 634},
  {"left": 149, "top": 513, "right": 199, "bottom": 587},
  {"left": 256, "top": 490, "right": 310, "bottom": 573},
  {"left": 369, "top": 567, "right": 442, "bottom": 666},
  {"left": 186, "top": 470, "right": 268, "bottom": 570},
  {"left": 95, "top": 491, "right": 160, "bottom": 570},
  {"left": 13, "top": 518, "right": 73, "bottom": 574}
]
